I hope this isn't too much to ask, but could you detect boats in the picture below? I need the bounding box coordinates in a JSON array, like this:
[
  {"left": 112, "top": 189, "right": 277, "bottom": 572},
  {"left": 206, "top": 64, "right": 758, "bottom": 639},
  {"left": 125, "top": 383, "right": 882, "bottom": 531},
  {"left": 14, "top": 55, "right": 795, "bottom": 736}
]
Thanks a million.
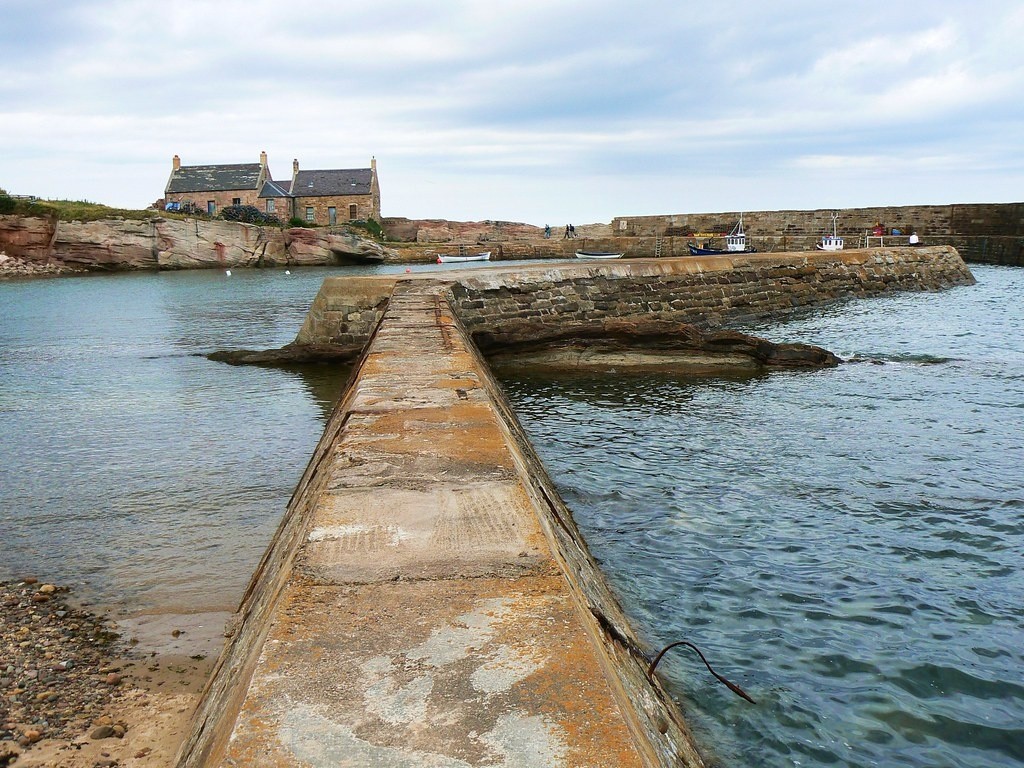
[
  {"left": 816, "top": 212, "right": 844, "bottom": 250},
  {"left": 438, "top": 251, "right": 492, "bottom": 263},
  {"left": 688, "top": 212, "right": 757, "bottom": 256},
  {"left": 574, "top": 251, "right": 625, "bottom": 259}
]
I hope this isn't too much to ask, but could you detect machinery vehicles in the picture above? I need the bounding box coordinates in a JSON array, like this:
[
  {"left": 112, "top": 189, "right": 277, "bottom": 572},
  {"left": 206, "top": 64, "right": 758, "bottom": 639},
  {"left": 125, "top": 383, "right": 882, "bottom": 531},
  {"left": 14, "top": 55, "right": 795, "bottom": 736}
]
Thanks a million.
[{"left": 166, "top": 201, "right": 196, "bottom": 216}]
